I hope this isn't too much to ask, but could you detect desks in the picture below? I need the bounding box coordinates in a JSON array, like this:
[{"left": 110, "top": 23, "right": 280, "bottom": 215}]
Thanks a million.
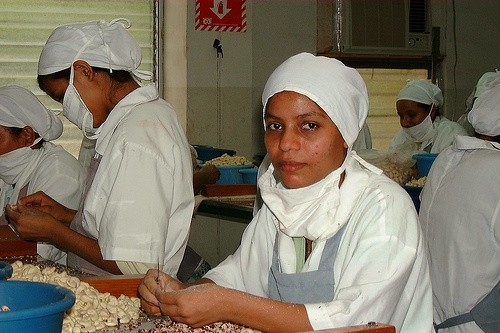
[{"left": 188, "top": 196, "right": 254, "bottom": 269}]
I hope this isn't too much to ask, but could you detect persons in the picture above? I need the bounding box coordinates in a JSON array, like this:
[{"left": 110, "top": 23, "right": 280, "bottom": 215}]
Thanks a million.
[
  {"left": 0, "top": 85, "right": 88, "bottom": 268},
  {"left": 6, "top": 17, "right": 194, "bottom": 280},
  {"left": 67, "top": 131, "right": 220, "bottom": 282},
  {"left": 417, "top": 69, "right": 500, "bottom": 333},
  {"left": 385, "top": 79, "right": 470, "bottom": 166},
  {"left": 457, "top": 86, "right": 476, "bottom": 137},
  {"left": 138, "top": 52, "right": 436, "bottom": 333}
]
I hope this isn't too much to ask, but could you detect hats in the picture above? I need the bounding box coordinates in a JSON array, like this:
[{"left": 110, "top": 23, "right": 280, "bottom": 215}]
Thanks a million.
[
  {"left": 472, "top": 69, "right": 500, "bottom": 98},
  {"left": 38, "top": 19, "right": 143, "bottom": 75},
  {"left": 467, "top": 86, "right": 500, "bottom": 137},
  {"left": 0, "top": 85, "right": 63, "bottom": 142},
  {"left": 262, "top": 51, "right": 369, "bottom": 149},
  {"left": 397, "top": 79, "right": 444, "bottom": 109}
]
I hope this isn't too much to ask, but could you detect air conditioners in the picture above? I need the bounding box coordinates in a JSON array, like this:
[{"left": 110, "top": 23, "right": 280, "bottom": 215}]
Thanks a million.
[{"left": 332, "top": 0, "right": 433, "bottom": 56}]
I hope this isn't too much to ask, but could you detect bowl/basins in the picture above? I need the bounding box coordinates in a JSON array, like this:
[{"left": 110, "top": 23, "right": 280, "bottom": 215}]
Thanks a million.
[
  {"left": 194, "top": 145, "right": 237, "bottom": 163},
  {"left": 197, "top": 164, "right": 255, "bottom": 184},
  {"left": 0, "top": 260, "right": 13, "bottom": 281},
  {"left": 237, "top": 168, "right": 258, "bottom": 184},
  {"left": 0, "top": 279, "right": 76, "bottom": 333},
  {"left": 415, "top": 153, "right": 439, "bottom": 178}
]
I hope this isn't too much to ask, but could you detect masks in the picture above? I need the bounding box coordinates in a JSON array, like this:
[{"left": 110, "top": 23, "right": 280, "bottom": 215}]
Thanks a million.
[
  {"left": 62, "top": 66, "right": 104, "bottom": 140},
  {"left": 401, "top": 103, "right": 434, "bottom": 143},
  {"left": 0, "top": 145, "right": 39, "bottom": 185},
  {"left": 257, "top": 155, "right": 350, "bottom": 241}
]
